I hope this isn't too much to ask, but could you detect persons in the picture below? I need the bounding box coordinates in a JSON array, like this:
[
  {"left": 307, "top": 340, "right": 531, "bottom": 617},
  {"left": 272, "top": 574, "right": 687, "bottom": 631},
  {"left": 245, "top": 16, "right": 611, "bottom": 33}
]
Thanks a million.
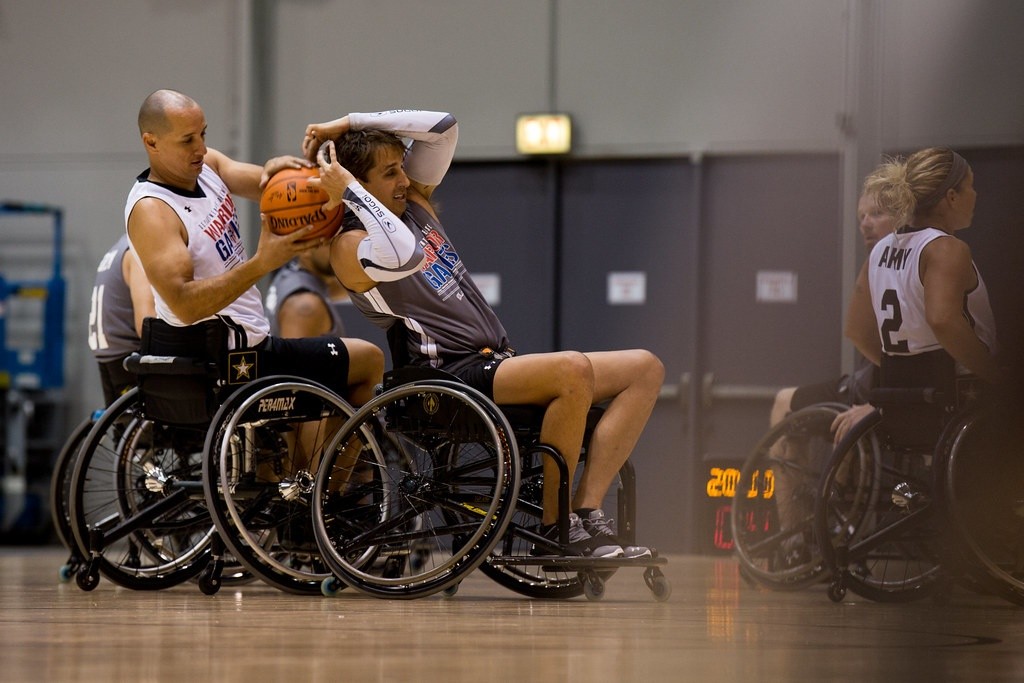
[
  {"left": 87, "top": 90, "right": 666, "bottom": 562},
  {"left": 765, "top": 144, "right": 1024, "bottom": 576}
]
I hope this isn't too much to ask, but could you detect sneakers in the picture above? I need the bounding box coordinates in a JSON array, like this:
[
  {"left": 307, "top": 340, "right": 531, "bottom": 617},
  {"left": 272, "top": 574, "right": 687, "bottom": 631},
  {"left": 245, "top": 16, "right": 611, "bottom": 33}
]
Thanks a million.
[
  {"left": 529, "top": 513, "right": 624, "bottom": 558},
  {"left": 574, "top": 506, "right": 652, "bottom": 558}
]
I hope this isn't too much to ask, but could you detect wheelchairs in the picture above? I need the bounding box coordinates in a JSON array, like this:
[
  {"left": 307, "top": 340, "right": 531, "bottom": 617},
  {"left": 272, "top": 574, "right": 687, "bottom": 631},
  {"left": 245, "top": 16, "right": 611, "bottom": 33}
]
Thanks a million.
[
  {"left": 46, "top": 313, "right": 672, "bottom": 609},
  {"left": 728, "top": 376, "right": 1023, "bottom": 611}
]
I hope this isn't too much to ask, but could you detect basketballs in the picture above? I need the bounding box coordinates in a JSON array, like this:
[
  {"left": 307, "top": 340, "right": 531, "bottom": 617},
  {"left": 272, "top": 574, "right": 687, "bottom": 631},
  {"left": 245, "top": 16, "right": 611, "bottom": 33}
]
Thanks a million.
[{"left": 260, "top": 164, "right": 340, "bottom": 246}]
